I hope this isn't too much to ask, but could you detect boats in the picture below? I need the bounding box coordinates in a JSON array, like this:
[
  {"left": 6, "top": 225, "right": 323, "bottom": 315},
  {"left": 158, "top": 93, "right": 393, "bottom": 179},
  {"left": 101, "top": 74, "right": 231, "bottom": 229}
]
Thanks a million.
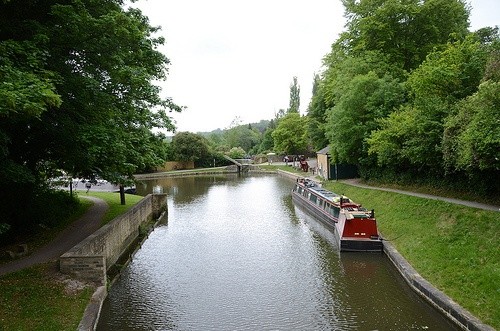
[
  {"left": 290, "top": 176, "right": 383, "bottom": 254},
  {"left": 118, "top": 182, "right": 136, "bottom": 194}
]
